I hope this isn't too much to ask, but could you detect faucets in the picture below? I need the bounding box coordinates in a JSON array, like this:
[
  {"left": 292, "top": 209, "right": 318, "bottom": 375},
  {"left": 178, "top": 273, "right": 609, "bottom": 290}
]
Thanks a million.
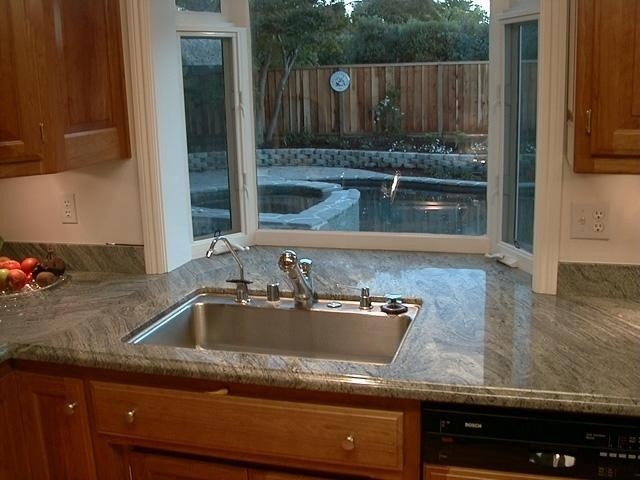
[
  {"left": 278, "top": 250, "right": 314, "bottom": 302},
  {"left": 206, "top": 237, "right": 244, "bottom": 279}
]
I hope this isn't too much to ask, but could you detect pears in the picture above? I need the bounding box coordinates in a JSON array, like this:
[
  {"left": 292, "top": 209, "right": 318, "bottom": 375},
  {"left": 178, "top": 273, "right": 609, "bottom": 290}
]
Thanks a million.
[
  {"left": 36, "top": 272, "right": 57, "bottom": 287},
  {"left": 39, "top": 258, "right": 65, "bottom": 276}
]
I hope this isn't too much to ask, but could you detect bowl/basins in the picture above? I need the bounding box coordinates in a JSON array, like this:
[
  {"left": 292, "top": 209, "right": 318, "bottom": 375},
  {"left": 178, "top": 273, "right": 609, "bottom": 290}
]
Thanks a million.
[{"left": 0, "top": 273, "right": 72, "bottom": 321}]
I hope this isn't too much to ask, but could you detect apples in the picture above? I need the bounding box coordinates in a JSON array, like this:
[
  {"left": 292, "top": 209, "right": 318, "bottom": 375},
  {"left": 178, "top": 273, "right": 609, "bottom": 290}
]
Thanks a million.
[{"left": 0, "top": 256, "right": 39, "bottom": 289}]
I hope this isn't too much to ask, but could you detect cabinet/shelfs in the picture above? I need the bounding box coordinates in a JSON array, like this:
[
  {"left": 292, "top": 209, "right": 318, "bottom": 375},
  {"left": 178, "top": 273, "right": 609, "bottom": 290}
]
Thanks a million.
[
  {"left": 0, "top": 0, "right": 130, "bottom": 178},
  {"left": 567, "top": 0, "right": 640, "bottom": 174},
  {"left": 90, "top": 362, "right": 421, "bottom": 480},
  {"left": 1, "top": 356, "right": 90, "bottom": 480}
]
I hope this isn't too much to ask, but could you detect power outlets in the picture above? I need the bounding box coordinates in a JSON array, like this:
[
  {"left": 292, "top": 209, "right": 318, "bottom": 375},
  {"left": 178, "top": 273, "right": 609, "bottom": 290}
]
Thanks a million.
[
  {"left": 572, "top": 200, "right": 611, "bottom": 240},
  {"left": 60, "top": 192, "right": 78, "bottom": 224}
]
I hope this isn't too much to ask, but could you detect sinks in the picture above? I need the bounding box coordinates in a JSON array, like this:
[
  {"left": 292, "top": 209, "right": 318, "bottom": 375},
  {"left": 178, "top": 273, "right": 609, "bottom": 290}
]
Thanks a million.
[{"left": 120, "top": 285, "right": 423, "bottom": 366}]
